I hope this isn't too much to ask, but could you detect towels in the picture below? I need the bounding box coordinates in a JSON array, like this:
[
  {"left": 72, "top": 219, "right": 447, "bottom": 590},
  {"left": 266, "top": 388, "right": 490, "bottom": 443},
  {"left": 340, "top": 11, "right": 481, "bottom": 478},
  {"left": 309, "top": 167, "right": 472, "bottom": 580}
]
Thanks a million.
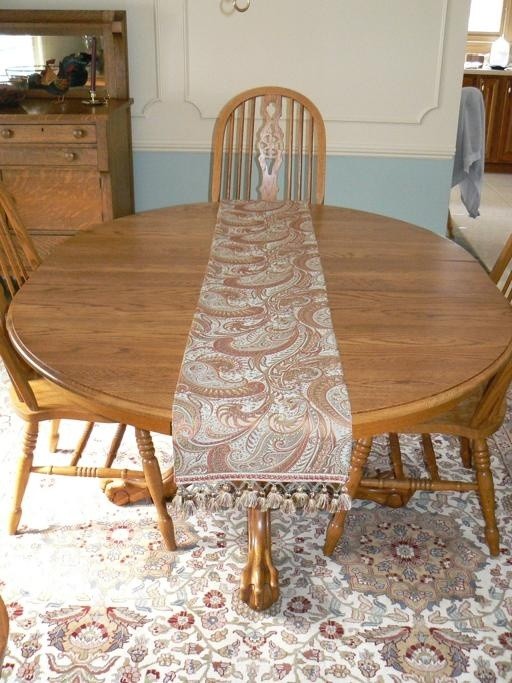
[{"left": 171, "top": 199, "right": 353, "bottom": 517}]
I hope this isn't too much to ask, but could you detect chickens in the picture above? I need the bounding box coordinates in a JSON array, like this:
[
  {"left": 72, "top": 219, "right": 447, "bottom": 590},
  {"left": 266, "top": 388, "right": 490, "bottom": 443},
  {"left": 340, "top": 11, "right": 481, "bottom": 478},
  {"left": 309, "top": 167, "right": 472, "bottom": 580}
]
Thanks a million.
[{"left": 39, "top": 53, "right": 87, "bottom": 104}]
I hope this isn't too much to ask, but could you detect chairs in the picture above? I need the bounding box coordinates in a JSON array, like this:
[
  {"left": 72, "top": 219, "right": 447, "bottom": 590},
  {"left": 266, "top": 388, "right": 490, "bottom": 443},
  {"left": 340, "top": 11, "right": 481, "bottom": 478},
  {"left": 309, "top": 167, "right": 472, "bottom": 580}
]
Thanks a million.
[
  {"left": 208, "top": 86, "right": 326, "bottom": 206},
  {"left": 2, "top": 189, "right": 178, "bottom": 554},
  {"left": 322, "top": 231, "right": 511, "bottom": 559}
]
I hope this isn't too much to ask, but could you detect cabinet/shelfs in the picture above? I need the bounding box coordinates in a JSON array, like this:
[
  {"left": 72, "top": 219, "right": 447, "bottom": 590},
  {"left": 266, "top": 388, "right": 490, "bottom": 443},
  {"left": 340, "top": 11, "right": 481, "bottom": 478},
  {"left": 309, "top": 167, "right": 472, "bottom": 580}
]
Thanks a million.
[
  {"left": 463, "top": 74, "right": 512, "bottom": 174},
  {"left": 0, "top": 8, "right": 133, "bottom": 280}
]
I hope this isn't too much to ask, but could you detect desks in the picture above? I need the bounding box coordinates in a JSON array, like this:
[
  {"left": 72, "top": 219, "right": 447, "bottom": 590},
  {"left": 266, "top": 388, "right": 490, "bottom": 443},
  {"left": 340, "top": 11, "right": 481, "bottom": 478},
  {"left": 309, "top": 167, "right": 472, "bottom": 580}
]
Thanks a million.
[{"left": 6, "top": 200, "right": 512, "bottom": 606}]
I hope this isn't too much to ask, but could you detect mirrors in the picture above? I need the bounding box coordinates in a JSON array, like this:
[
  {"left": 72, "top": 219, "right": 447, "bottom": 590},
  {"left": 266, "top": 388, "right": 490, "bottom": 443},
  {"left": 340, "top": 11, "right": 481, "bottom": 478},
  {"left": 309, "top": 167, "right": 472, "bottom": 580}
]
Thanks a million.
[{"left": 0, "top": 34, "right": 104, "bottom": 88}]
